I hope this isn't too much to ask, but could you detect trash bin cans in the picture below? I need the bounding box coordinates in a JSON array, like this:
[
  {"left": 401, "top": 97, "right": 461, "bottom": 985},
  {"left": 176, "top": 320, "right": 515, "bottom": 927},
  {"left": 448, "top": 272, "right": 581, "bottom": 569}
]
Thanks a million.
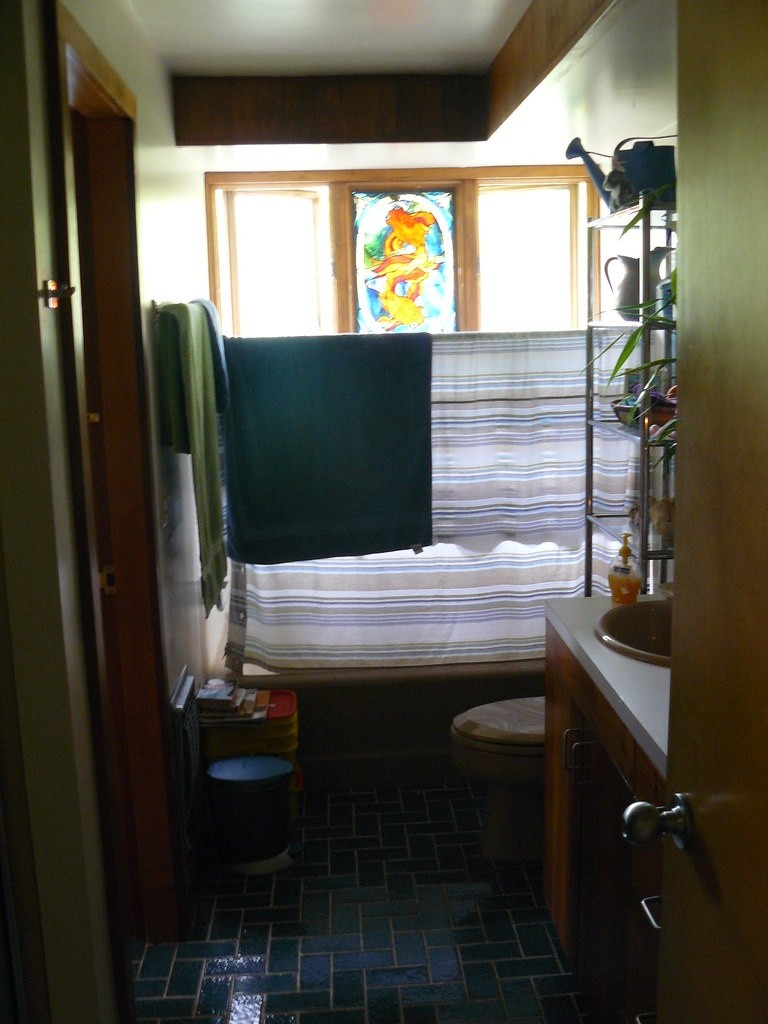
[{"left": 209, "top": 756, "right": 297, "bottom": 869}]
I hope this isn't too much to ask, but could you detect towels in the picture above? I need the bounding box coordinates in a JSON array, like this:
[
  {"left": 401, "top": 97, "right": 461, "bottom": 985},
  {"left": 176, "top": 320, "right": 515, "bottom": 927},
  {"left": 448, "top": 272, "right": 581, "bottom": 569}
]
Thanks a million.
[
  {"left": 223, "top": 331, "right": 433, "bottom": 565},
  {"left": 154, "top": 300, "right": 230, "bottom": 619}
]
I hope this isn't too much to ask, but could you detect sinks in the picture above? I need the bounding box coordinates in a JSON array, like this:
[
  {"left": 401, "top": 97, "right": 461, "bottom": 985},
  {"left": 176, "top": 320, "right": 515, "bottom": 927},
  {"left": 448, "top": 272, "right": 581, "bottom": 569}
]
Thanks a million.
[{"left": 594, "top": 600, "right": 672, "bottom": 667}]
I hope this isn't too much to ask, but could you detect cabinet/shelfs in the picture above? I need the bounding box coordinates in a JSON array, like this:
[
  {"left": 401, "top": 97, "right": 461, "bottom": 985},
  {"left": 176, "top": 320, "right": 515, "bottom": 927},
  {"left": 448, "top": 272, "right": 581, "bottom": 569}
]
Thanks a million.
[
  {"left": 545, "top": 638, "right": 664, "bottom": 1024},
  {"left": 581, "top": 188, "right": 676, "bottom": 597}
]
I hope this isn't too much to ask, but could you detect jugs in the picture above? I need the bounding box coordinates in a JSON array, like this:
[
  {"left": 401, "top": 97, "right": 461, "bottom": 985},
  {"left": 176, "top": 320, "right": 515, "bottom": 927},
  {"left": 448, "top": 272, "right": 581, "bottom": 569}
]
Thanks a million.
[{"left": 606, "top": 247, "right": 678, "bottom": 321}]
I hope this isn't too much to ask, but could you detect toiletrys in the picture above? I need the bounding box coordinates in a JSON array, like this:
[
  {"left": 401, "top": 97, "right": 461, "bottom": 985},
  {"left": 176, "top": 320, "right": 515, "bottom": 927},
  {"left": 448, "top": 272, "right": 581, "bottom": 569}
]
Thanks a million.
[{"left": 608, "top": 533, "right": 642, "bottom": 604}]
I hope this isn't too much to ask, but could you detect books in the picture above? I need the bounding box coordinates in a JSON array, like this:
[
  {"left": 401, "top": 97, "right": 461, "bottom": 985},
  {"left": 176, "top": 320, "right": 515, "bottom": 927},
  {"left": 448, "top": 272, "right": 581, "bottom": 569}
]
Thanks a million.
[{"left": 196, "top": 678, "right": 271, "bottom": 723}]
